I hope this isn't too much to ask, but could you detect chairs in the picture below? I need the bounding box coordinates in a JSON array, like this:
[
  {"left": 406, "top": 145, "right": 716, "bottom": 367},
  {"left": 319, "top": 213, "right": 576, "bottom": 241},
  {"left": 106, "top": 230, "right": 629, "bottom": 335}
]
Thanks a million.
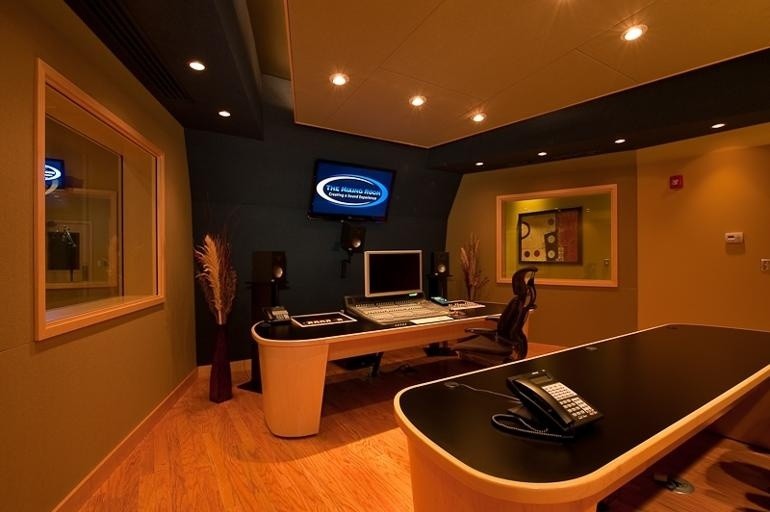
[{"left": 450, "top": 265, "right": 537, "bottom": 370}]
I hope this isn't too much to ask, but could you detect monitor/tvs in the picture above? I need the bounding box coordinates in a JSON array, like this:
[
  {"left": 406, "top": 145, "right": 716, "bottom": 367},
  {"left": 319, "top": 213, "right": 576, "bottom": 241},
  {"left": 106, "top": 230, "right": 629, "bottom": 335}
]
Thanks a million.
[
  {"left": 307, "top": 158, "right": 396, "bottom": 222},
  {"left": 48, "top": 231, "right": 79, "bottom": 270},
  {"left": 364, "top": 250, "right": 423, "bottom": 298}
]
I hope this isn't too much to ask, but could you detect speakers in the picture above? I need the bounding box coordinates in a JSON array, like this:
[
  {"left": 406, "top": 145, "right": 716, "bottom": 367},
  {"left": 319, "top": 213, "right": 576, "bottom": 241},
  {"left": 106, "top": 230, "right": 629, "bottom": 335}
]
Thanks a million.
[
  {"left": 430, "top": 250, "right": 449, "bottom": 276},
  {"left": 340, "top": 222, "right": 368, "bottom": 253},
  {"left": 252, "top": 251, "right": 287, "bottom": 284}
]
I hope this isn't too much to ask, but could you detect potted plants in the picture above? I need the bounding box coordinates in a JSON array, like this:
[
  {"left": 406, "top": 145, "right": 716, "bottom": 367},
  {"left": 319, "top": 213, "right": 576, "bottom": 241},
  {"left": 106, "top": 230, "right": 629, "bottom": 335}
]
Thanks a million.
[{"left": 192, "top": 224, "right": 238, "bottom": 404}]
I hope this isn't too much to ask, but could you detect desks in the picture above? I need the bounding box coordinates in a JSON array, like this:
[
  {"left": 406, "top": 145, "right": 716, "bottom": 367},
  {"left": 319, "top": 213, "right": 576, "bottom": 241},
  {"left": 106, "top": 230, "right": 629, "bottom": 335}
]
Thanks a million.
[
  {"left": 392, "top": 322, "right": 770, "bottom": 511},
  {"left": 252, "top": 297, "right": 530, "bottom": 440}
]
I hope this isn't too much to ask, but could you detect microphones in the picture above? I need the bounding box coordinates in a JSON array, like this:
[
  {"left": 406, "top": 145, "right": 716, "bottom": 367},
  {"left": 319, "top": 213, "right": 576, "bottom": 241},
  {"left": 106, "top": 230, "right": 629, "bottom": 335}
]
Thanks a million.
[{"left": 64, "top": 230, "right": 77, "bottom": 248}]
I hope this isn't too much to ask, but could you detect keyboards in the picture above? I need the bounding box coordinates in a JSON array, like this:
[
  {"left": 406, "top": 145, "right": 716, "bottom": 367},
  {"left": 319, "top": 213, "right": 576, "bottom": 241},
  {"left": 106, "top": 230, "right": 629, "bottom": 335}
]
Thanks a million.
[{"left": 410, "top": 315, "right": 454, "bottom": 324}]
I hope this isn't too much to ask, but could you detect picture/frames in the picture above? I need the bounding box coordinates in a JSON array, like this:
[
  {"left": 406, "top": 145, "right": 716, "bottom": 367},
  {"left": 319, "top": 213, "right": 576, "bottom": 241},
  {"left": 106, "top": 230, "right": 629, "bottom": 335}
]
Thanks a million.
[
  {"left": 46, "top": 219, "right": 93, "bottom": 289},
  {"left": 516, "top": 205, "right": 583, "bottom": 265}
]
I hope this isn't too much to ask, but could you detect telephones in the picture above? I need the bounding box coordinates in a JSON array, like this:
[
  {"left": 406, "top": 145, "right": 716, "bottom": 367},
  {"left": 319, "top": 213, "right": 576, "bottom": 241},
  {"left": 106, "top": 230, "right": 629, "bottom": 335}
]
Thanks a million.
[
  {"left": 504, "top": 367, "right": 605, "bottom": 433},
  {"left": 262, "top": 305, "right": 291, "bottom": 323}
]
